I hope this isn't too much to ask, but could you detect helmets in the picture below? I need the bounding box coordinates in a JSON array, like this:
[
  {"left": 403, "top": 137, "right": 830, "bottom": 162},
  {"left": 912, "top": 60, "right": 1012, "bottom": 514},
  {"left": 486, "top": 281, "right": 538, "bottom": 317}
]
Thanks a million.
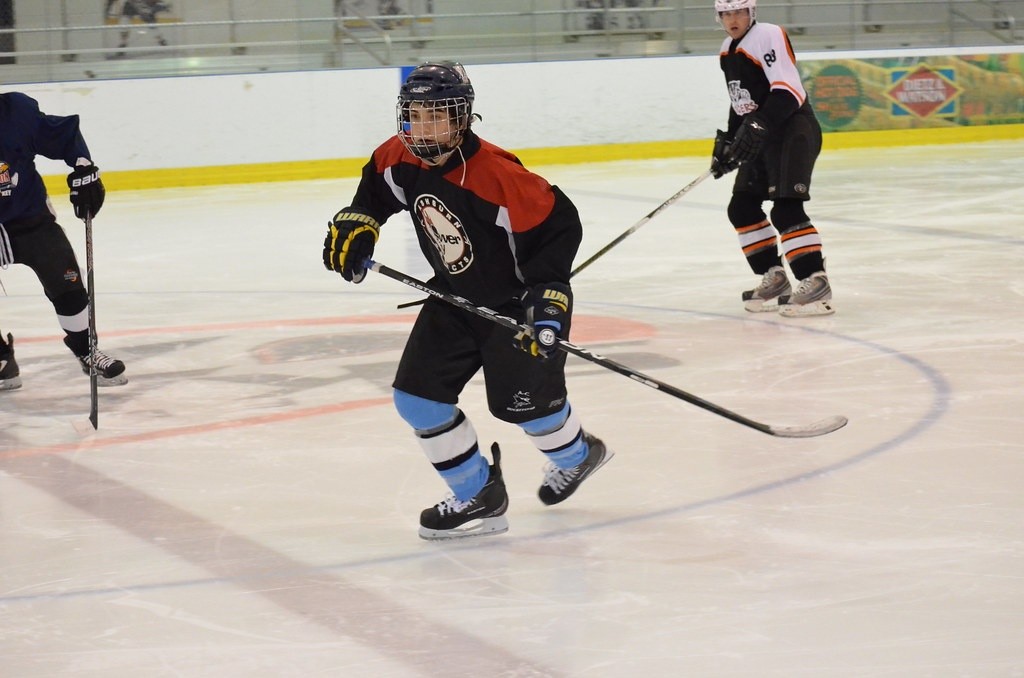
[
  {"left": 401, "top": 62, "right": 474, "bottom": 128},
  {"left": 714, "top": 1, "right": 757, "bottom": 27}
]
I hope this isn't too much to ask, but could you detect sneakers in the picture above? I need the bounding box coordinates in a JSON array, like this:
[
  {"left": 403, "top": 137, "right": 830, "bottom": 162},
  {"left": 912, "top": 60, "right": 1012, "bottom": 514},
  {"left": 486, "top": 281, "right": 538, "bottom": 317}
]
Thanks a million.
[
  {"left": 742, "top": 271, "right": 791, "bottom": 312},
  {"left": 0, "top": 347, "right": 22, "bottom": 392},
  {"left": 420, "top": 465, "right": 510, "bottom": 540},
  {"left": 79, "top": 348, "right": 129, "bottom": 387},
  {"left": 539, "top": 431, "right": 616, "bottom": 510},
  {"left": 779, "top": 276, "right": 835, "bottom": 318}
]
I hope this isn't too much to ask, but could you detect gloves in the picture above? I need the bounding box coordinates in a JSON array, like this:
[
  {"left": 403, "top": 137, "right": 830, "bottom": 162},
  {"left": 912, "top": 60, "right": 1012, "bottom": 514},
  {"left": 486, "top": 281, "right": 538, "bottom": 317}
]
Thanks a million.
[
  {"left": 710, "top": 129, "right": 742, "bottom": 179},
  {"left": 323, "top": 207, "right": 380, "bottom": 283},
  {"left": 509, "top": 283, "right": 573, "bottom": 365},
  {"left": 68, "top": 167, "right": 106, "bottom": 220},
  {"left": 730, "top": 114, "right": 769, "bottom": 163}
]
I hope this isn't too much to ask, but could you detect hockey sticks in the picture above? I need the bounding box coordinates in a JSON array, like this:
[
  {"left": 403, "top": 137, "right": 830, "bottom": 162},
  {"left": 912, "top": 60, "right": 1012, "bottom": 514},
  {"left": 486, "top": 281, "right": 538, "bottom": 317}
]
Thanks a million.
[
  {"left": 83, "top": 210, "right": 101, "bottom": 434},
  {"left": 566, "top": 159, "right": 728, "bottom": 279},
  {"left": 366, "top": 259, "right": 850, "bottom": 441}
]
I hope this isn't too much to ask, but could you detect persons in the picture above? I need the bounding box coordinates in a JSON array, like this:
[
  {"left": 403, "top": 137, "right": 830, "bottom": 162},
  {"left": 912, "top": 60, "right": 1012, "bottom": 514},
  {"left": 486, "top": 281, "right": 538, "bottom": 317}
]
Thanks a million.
[
  {"left": 708, "top": 0, "right": 837, "bottom": 317},
  {"left": 322, "top": 59, "right": 616, "bottom": 543},
  {"left": 0, "top": 91, "right": 129, "bottom": 391}
]
